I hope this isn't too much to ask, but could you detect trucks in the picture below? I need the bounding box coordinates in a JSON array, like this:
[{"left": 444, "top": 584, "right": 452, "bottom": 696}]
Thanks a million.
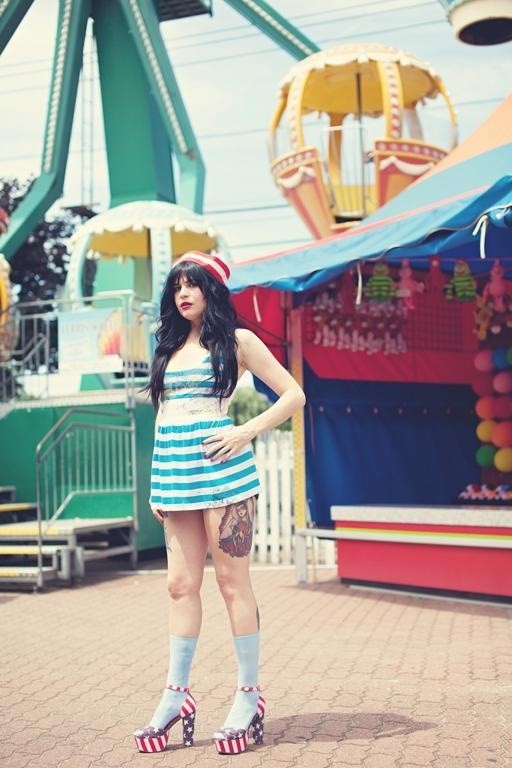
[{"left": 471, "top": 343, "right": 512, "bottom": 473}]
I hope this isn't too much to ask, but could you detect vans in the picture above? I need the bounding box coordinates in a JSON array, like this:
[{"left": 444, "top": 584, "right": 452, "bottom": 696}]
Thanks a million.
[
  {"left": 132, "top": 682, "right": 198, "bottom": 754},
  {"left": 212, "top": 681, "right": 266, "bottom": 755}
]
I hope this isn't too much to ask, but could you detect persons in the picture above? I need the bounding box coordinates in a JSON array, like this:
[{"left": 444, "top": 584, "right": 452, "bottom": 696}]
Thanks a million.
[{"left": 132, "top": 250, "right": 305, "bottom": 756}]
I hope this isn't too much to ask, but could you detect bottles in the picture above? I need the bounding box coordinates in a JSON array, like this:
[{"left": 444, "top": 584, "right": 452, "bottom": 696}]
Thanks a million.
[{"left": 171, "top": 249, "right": 230, "bottom": 286}]
